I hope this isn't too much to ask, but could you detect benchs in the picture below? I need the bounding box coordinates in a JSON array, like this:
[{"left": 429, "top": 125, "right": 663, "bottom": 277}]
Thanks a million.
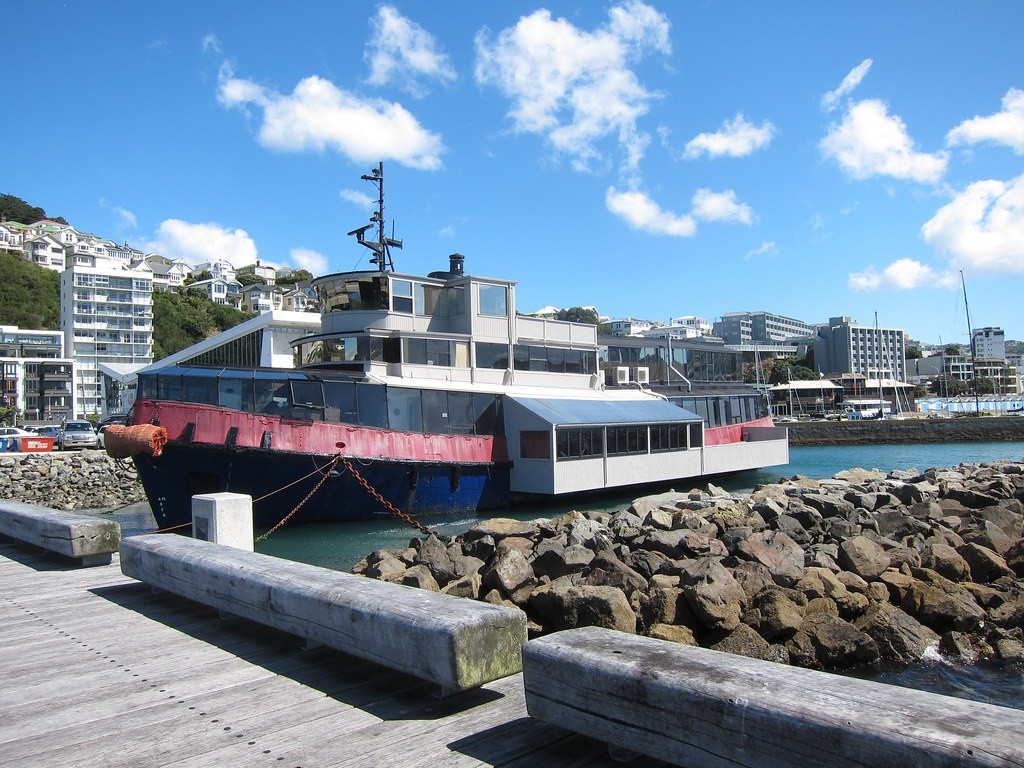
[
  {"left": 120, "top": 532, "right": 528, "bottom": 698},
  {"left": 520, "top": 625, "right": 1024, "bottom": 768},
  {"left": 0, "top": 498, "right": 120, "bottom": 568}
]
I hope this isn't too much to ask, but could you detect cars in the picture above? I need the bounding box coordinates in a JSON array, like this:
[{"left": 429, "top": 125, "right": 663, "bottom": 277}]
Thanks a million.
[
  {"left": 15, "top": 423, "right": 62, "bottom": 447},
  {"left": 0, "top": 427, "right": 41, "bottom": 439},
  {"left": 98, "top": 425, "right": 127, "bottom": 451}
]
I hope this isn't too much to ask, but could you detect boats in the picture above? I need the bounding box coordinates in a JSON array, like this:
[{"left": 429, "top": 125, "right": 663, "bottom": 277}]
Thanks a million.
[{"left": 98, "top": 160, "right": 791, "bottom": 523}]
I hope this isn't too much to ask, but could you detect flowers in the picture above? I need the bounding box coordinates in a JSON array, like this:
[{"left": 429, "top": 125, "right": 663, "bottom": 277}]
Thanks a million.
[{"left": 743, "top": 432, "right": 749, "bottom": 436}]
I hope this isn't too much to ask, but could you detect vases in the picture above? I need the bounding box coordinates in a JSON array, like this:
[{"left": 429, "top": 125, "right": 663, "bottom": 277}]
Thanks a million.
[{"left": 745, "top": 436, "right": 750, "bottom": 442}]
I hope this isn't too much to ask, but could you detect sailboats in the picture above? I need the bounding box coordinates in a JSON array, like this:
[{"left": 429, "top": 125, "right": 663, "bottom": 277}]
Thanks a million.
[{"left": 842, "top": 269, "right": 1024, "bottom": 420}]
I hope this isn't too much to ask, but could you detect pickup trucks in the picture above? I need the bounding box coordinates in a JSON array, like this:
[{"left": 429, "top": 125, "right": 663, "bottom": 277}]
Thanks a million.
[{"left": 56, "top": 419, "right": 99, "bottom": 452}]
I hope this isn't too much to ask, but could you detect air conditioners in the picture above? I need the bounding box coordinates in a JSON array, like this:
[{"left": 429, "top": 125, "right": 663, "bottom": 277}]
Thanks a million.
[
  {"left": 611, "top": 366, "right": 629, "bottom": 385},
  {"left": 633, "top": 366, "right": 650, "bottom": 385}
]
